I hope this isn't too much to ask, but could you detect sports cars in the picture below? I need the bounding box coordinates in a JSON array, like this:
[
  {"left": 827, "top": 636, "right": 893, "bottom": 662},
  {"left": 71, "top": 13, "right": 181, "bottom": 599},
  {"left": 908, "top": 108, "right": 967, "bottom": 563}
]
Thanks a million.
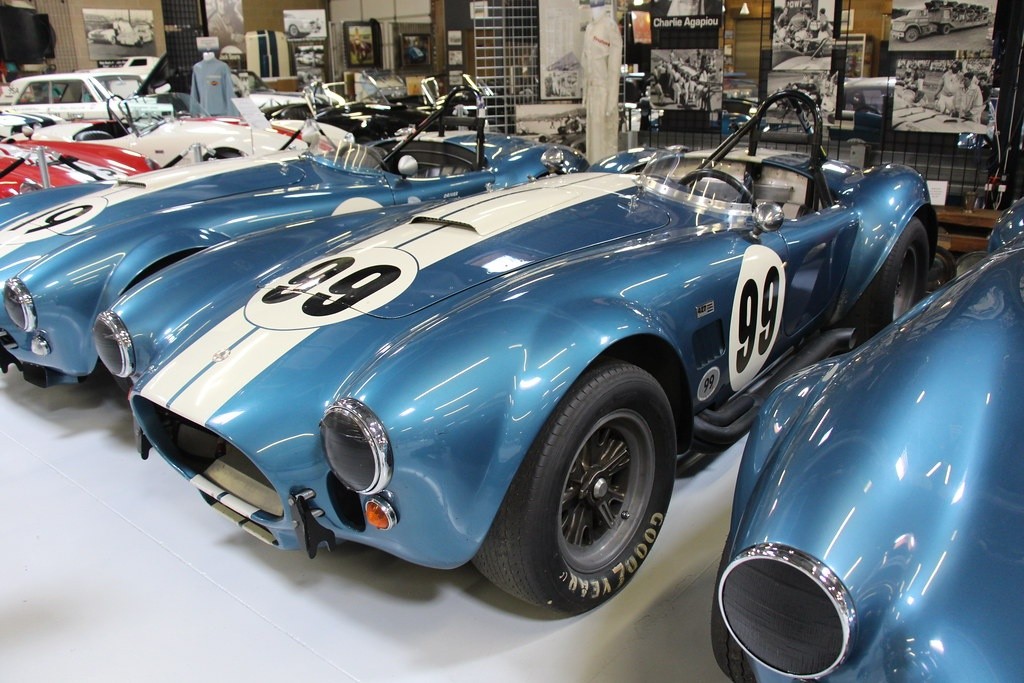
[
  {"left": 0, "top": 57, "right": 591, "bottom": 400},
  {"left": 93, "top": 85, "right": 938, "bottom": 620},
  {"left": 709, "top": 191, "right": 1022, "bottom": 683}
]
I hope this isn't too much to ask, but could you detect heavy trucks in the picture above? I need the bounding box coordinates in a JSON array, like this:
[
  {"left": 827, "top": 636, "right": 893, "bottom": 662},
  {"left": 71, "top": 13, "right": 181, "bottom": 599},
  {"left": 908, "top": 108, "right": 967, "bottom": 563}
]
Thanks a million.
[{"left": 891, "top": 7, "right": 990, "bottom": 42}]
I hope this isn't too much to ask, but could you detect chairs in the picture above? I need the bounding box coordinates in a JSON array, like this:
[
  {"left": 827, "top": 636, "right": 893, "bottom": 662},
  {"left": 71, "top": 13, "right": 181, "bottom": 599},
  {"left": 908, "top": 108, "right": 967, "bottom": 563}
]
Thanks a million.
[
  {"left": 73, "top": 131, "right": 114, "bottom": 141},
  {"left": 342, "top": 146, "right": 387, "bottom": 169}
]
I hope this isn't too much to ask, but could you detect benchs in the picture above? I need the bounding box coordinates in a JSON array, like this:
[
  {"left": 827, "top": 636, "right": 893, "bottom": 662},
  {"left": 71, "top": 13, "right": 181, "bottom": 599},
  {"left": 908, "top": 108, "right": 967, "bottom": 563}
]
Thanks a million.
[{"left": 279, "top": 104, "right": 332, "bottom": 121}]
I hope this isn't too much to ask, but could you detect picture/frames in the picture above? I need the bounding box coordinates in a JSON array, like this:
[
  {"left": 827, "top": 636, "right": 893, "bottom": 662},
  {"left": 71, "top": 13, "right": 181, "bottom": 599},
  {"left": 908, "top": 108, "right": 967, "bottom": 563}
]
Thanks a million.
[
  {"left": 344, "top": 22, "right": 377, "bottom": 67},
  {"left": 399, "top": 32, "right": 433, "bottom": 66}
]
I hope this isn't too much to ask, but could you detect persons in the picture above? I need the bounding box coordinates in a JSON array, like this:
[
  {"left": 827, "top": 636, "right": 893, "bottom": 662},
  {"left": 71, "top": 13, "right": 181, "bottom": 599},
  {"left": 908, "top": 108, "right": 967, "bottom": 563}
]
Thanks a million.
[
  {"left": 189, "top": 52, "right": 239, "bottom": 117},
  {"left": 895, "top": 58, "right": 992, "bottom": 124},
  {"left": 652, "top": 49, "right": 716, "bottom": 107},
  {"left": 844, "top": 90, "right": 880, "bottom": 114},
  {"left": 775, "top": 4, "right": 829, "bottom": 42},
  {"left": 535, "top": 113, "right": 582, "bottom": 133},
  {"left": 776, "top": 78, "right": 822, "bottom": 115}
]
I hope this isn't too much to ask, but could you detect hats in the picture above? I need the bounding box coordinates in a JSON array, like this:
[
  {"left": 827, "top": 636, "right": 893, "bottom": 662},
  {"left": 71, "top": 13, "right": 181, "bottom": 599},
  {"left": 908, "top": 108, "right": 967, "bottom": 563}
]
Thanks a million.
[
  {"left": 949, "top": 61, "right": 962, "bottom": 70},
  {"left": 804, "top": 4, "right": 812, "bottom": 8}
]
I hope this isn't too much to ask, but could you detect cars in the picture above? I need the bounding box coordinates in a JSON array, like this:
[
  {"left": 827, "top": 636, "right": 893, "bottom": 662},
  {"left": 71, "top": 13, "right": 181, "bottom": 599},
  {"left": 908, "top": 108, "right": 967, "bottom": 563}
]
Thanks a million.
[
  {"left": 87, "top": 21, "right": 154, "bottom": 48},
  {"left": 408, "top": 45, "right": 428, "bottom": 62},
  {"left": 283, "top": 14, "right": 323, "bottom": 38}
]
